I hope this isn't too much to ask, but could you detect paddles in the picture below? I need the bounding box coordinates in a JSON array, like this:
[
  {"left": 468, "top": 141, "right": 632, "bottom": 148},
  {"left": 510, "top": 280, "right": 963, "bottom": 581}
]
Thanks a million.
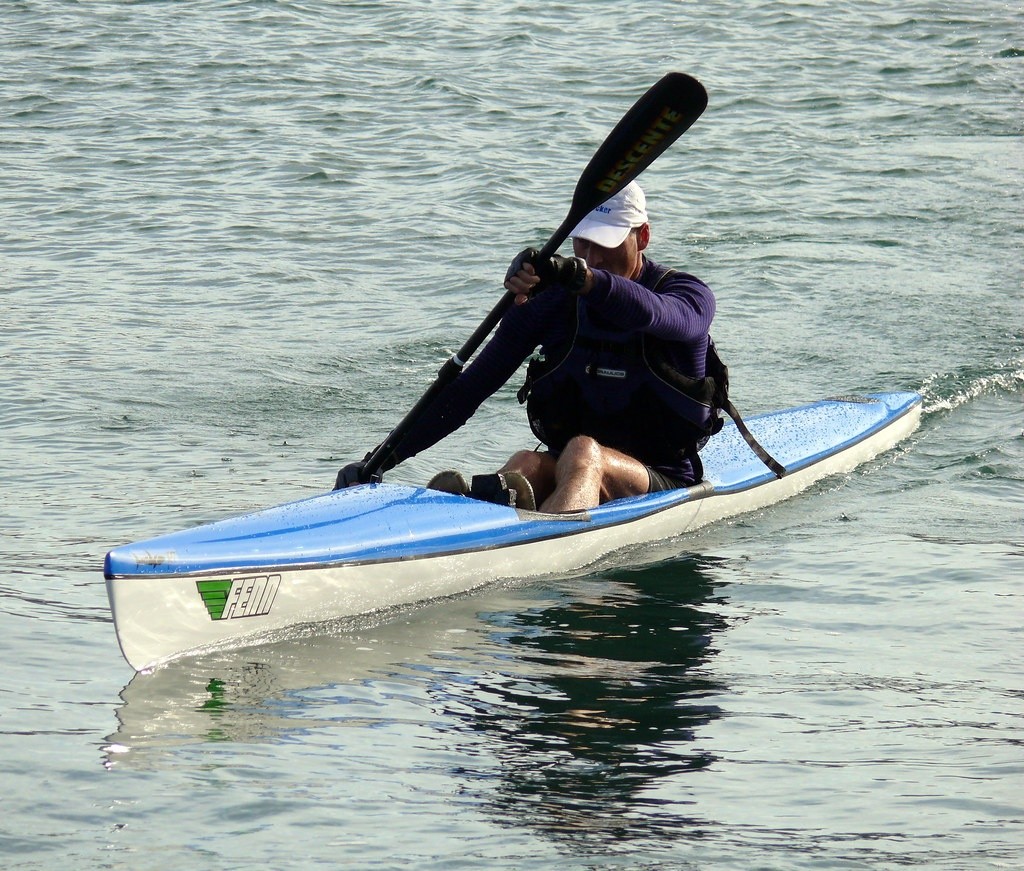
[{"left": 359, "top": 72, "right": 708, "bottom": 483}]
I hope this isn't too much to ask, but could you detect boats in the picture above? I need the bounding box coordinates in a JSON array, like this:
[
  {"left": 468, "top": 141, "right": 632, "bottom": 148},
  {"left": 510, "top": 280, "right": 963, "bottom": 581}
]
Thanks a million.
[{"left": 102, "top": 384, "right": 923, "bottom": 670}]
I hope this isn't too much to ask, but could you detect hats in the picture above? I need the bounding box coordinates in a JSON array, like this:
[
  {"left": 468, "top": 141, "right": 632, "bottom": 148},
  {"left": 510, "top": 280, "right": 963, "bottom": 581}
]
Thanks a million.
[{"left": 567, "top": 179, "right": 648, "bottom": 248}]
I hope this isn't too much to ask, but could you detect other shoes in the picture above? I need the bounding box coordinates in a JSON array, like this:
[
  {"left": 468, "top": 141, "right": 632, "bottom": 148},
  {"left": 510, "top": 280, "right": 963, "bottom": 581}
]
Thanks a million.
[
  {"left": 425, "top": 470, "right": 470, "bottom": 498},
  {"left": 502, "top": 471, "right": 536, "bottom": 511}
]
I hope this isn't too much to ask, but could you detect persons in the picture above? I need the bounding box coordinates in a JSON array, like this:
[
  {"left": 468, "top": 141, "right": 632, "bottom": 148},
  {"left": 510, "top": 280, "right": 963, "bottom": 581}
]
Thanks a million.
[{"left": 425, "top": 180, "right": 716, "bottom": 513}]
